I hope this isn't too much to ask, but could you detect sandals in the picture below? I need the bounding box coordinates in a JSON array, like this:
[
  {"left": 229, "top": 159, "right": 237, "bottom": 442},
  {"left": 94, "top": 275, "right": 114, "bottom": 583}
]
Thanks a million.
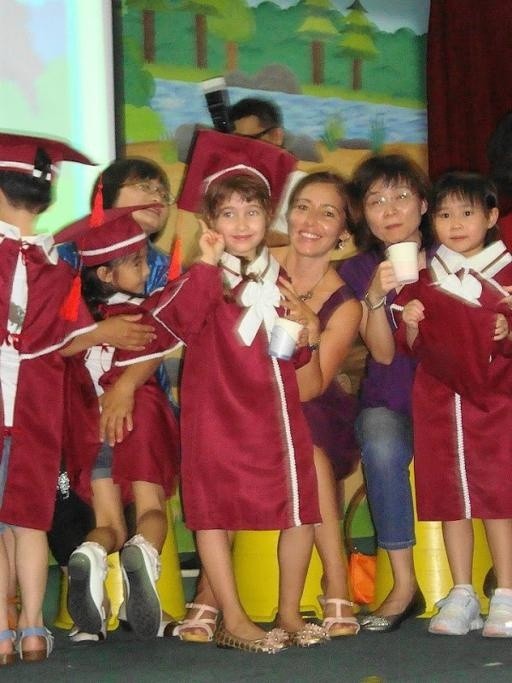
[{"left": 0, "top": 627, "right": 55, "bottom": 665}]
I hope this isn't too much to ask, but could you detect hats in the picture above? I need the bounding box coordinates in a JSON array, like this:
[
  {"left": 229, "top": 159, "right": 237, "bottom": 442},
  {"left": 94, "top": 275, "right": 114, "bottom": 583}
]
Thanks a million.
[
  {"left": 168, "top": 129, "right": 294, "bottom": 283},
  {"left": 54, "top": 204, "right": 165, "bottom": 323},
  {"left": 0, "top": 133, "right": 99, "bottom": 182}
]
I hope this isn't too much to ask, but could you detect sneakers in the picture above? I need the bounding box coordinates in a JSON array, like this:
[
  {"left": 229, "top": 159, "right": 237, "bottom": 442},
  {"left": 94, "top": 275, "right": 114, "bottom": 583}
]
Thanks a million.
[{"left": 359, "top": 586, "right": 512, "bottom": 637}]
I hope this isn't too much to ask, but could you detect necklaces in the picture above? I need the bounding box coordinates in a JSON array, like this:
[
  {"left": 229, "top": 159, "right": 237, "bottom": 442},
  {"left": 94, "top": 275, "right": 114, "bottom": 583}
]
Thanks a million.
[{"left": 279, "top": 254, "right": 333, "bottom": 303}]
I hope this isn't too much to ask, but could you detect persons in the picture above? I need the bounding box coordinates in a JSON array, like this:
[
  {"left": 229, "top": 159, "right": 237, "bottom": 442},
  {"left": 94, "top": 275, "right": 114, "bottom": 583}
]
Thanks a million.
[
  {"left": 1, "top": 169, "right": 99, "bottom": 667},
  {"left": 391, "top": 164, "right": 512, "bottom": 640},
  {"left": 181, "top": 172, "right": 364, "bottom": 642},
  {"left": 151, "top": 163, "right": 327, "bottom": 655},
  {"left": 334, "top": 152, "right": 437, "bottom": 633},
  {"left": 225, "top": 98, "right": 309, "bottom": 247},
  {"left": 49, "top": 154, "right": 189, "bottom": 644},
  {"left": 43, "top": 242, "right": 179, "bottom": 643}
]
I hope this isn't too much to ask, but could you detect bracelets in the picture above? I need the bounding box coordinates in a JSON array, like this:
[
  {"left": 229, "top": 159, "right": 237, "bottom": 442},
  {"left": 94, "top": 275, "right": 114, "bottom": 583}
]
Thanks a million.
[{"left": 310, "top": 339, "right": 321, "bottom": 355}]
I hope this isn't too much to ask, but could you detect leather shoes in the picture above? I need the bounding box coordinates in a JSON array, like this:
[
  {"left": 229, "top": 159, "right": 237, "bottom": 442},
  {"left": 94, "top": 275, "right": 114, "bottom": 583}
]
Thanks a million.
[
  {"left": 68, "top": 535, "right": 181, "bottom": 642},
  {"left": 180, "top": 596, "right": 361, "bottom": 651}
]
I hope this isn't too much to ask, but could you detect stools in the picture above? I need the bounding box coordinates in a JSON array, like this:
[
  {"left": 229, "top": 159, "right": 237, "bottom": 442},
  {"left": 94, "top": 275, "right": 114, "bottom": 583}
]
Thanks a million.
[
  {"left": 368, "top": 454, "right": 497, "bottom": 617},
  {"left": 53, "top": 497, "right": 187, "bottom": 631},
  {"left": 232, "top": 527, "right": 362, "bottom": 622}
]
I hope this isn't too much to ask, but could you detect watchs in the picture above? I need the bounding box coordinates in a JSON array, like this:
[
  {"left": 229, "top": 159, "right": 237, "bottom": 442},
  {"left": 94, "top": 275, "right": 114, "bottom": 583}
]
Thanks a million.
[{"left": 364, "top": 292, "right": 388, "bottom": 314}]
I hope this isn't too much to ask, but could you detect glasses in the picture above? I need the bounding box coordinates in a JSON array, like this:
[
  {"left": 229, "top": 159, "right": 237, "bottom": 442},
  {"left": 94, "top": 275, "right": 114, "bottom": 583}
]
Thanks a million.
[
  {"left": 365, "top": 190, "right": 417, "bottom": 212},
  {"left": 119, "top": 181, "right": 174, "bottom": 203}
]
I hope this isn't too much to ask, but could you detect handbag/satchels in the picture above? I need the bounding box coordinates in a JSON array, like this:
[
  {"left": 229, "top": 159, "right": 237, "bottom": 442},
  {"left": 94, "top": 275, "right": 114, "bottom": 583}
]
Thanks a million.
[{"left": 349, "top": 551, "right": 377, "bottom": 604}]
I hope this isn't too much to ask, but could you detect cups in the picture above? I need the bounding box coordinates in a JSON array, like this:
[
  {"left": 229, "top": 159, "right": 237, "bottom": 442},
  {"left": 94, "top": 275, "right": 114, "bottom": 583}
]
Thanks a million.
[
  {"left": 386, "top": 241, "right": 419, "bottom": 284},
  {"left": 267, "top": 317, "right": 303, "bottom": 362}
]
